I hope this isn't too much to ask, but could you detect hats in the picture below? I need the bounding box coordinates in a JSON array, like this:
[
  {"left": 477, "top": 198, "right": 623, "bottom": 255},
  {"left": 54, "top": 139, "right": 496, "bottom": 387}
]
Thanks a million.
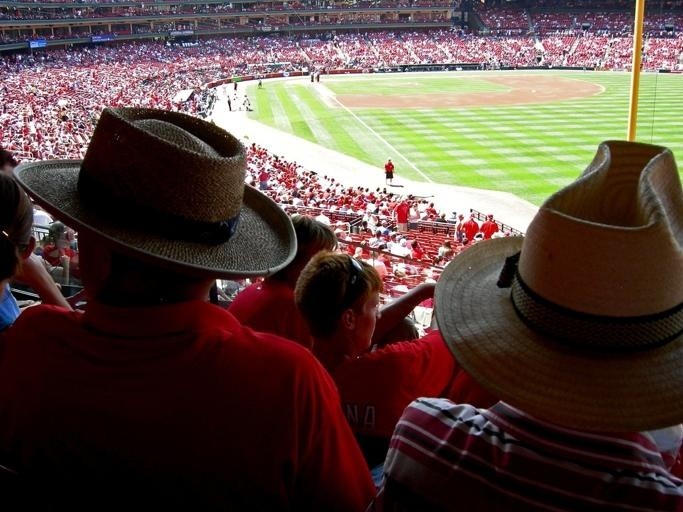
[
  {"left": 11, "top": 107, "right": 299, "bottom": 279},
  {"left": 433, "top": 140, "right": 682, "bottom": 436}
]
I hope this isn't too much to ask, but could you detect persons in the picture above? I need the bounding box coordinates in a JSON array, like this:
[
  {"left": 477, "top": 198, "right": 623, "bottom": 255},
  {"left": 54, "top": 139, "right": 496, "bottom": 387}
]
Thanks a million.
[
  {"left": 294, "top": 252, "right": 455, "bottom": 468},
  {"left": 1, "top": 106, "right": 379, "bottom": 512},
  {"left": 1, "top": 0, "right": 683, "bottom": 350},
  {"left": 360, "top": 138, "right": 683, "bottom": 512}
]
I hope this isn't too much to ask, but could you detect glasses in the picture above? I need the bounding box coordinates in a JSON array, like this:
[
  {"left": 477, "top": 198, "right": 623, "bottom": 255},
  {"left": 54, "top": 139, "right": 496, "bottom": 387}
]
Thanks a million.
[{"left": 345, "top": 254, "right": 364, "bottom": 292}]
